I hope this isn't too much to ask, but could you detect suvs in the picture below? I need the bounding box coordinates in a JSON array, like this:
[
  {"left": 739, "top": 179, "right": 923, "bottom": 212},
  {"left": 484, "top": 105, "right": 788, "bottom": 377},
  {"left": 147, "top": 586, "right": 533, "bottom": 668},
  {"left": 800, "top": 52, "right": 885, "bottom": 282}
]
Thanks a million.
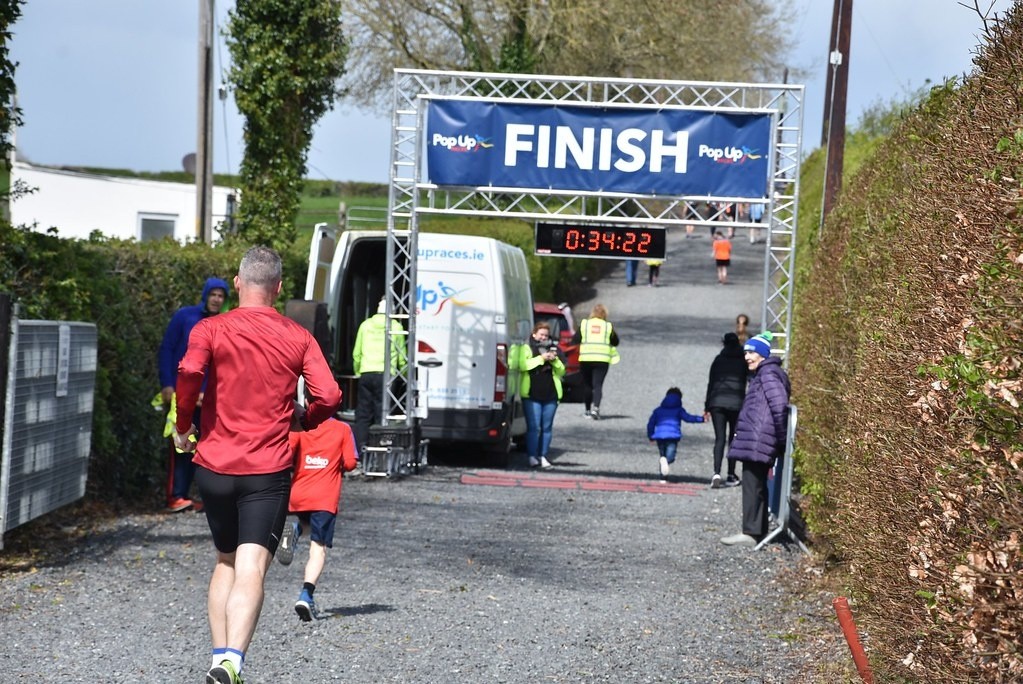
[{"left": 533, "top": 300, "right": 581, "bottom": 384}]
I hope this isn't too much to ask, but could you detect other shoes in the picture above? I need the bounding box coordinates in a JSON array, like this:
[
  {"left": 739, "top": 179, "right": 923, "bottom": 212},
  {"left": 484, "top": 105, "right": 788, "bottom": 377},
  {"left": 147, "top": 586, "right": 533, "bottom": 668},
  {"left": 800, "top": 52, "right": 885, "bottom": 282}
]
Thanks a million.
[
  {"left": 344, "top": 462, "right": 363, "bottom": 476},
  {"left": 720, "top": 534, "right": 761, "bottom": 546}
]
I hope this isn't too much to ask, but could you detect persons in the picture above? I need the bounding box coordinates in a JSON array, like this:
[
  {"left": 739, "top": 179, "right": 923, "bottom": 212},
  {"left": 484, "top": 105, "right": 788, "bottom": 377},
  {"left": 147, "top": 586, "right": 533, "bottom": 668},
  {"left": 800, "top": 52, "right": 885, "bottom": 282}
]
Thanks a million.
[
  {"left": 647, "top": 387, "right": 709, "bottom": 485},
  {"left": 157, "top": 278, "right": 230, "bottom": 512},
  {"left": 518, "top": 321, "right": 565, "bottom": 468},
  {"left": 573, "top": 304, "right": 619, "bottom": 420},
  {"left": 173, "top": 246, "right": 342, "bottom": 684},
  {"left": 344, "top": 299, "right": 409, "bottom": 480},
  {"left": 734, "top": 314, "right": 754, "bottom": 347},
  {"left": 683, "top": 200, "right": 765, "bottom": 244},
  {"left": 710, "top": 231, "right": 731, "bottom": 285},
  {"left": 720, "top": 331, "right": 792, "bottom": 547},
  {"left": 276, "top": 374, "right": 359, "bottom": 621},
  {"left": 626, "top": 260, "right": 639, "bottom": 285},
  {"left": 644, "top": 261, "right": 663, "bottom": 286},
  {"left": 704, "top": 332, "right": 756, "bottom": 488}
]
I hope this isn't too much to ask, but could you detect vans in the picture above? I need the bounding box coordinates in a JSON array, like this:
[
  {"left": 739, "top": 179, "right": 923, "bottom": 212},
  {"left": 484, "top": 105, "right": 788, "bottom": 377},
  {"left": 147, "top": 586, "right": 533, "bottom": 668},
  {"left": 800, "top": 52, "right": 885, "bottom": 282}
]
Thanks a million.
[{"left": 300, "top": 221, "right": 538, "bottom": 459}]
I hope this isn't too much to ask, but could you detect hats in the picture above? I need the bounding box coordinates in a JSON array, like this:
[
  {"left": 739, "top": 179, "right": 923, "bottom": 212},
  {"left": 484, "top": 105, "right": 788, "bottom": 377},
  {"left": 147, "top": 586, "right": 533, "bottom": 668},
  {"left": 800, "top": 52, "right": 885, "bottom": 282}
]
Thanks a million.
[{"left": 744, "top": 330, "right": 773, "bottom": 358}]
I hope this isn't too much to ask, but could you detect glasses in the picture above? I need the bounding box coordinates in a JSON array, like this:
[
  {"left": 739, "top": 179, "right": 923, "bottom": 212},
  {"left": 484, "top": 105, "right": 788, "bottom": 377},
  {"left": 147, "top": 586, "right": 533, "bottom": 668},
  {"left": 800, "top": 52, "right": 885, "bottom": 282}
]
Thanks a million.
[{"left": 737, "top": 321, "right": 748, "bottom": 327}]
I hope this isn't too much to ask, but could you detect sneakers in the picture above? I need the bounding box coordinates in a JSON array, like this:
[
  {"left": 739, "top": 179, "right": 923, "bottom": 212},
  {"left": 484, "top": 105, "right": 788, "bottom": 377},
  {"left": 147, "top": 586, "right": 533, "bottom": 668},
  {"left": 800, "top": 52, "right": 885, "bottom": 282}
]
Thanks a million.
[
  {"left": 528, "top": 457, "right": 554, "bottom": 472},
  {"left": 709, "top": 474, "right": 722, "bottom": 489},
  {"left": 295, "top": 590, "right": 319, "bottom": 622},
  {"left": 583, "top": 411, "right": 602, "bottom": 421},
  {"left": 725, "top": 475, "right": 741, "bottom": 486},
  {"left": 660, "top": 456, "right": 670, "bottom": 475},
  {"left": 275, "top": 515, "right": 302, "bottom": 565},
  {"left": 164, "top": 499, "right": 203, "bottom": 512},
  {"left": 206, "top": 661, "right": 244, "bottom": 684}
]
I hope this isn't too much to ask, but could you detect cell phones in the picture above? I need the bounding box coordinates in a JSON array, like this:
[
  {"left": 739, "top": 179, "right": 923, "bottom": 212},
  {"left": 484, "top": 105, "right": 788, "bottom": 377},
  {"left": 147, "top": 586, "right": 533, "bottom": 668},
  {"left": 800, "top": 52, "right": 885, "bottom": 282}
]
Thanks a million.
[{"left": 550, "top": 347, "right": 557, "bottom": 354}]
